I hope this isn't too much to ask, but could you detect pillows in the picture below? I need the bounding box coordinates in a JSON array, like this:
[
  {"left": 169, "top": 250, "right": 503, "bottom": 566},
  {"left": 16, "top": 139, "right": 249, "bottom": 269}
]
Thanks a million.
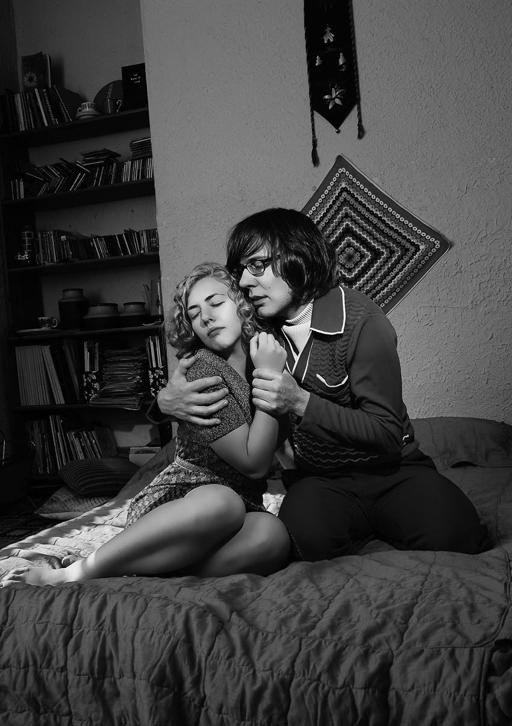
[
  {"left": 33, "top": 457, "right": 139, "bottom": 522},
  {"left": 409, "top": 417, "right": 512, "bottom": 472}
]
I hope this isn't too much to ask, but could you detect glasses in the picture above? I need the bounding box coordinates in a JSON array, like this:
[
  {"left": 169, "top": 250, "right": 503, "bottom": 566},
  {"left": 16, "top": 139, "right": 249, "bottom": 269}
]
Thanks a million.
[{"left": 232, "top": 254, "right": 280, "bottom": 277}]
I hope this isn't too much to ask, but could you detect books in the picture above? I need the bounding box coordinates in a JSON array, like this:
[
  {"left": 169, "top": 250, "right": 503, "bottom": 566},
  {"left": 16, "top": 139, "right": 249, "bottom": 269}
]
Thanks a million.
[{"left": 8, "top": 49, "right": 168, "bottom": 479}]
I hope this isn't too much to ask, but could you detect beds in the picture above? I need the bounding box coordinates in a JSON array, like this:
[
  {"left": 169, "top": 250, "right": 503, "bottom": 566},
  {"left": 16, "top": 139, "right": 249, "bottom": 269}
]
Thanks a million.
[{"left": 0, "top": 416, "right": 510, "bottom": 720}]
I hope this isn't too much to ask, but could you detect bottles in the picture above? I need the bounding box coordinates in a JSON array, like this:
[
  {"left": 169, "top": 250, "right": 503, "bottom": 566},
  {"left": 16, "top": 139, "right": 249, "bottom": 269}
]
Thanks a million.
[
  {"left": 57, "top": 287, "right": 146, "bottom": 331},
  {"left": 13, "top": 231, "right": 36, "bottom": 267}
]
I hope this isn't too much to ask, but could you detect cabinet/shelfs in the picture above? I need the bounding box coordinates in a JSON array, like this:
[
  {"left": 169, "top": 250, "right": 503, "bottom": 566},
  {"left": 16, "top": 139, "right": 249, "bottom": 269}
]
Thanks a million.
[{"left": 1, "top": 107, "right": 173, "bottom": 498}]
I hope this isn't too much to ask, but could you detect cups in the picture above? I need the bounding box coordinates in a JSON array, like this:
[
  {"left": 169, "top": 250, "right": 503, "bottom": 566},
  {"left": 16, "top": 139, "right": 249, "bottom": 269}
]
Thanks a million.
[
  {"left": 38, "top": 316, "right": 58, "bottom": 329},
  {"left": 103, "top": 95, "right": 123, "bottom": 114},
  {"left": 77, "top": 101, "right": 97, "bottom": 113}
]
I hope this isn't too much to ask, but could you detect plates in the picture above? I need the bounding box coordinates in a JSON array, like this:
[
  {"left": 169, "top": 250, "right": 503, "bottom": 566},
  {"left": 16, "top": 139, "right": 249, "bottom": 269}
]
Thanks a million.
[{"left": 75, "top": 111, "right": 99, "bottom": 119}]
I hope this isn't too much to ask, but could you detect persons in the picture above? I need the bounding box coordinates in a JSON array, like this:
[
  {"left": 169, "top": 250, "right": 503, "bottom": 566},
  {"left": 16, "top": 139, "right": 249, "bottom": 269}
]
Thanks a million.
[
  {"left": 144, "top": 206, "right": 490, "bottom": 554},
  {"left": 21, "top": 261, "right": 293, "bottom": 587}
]
